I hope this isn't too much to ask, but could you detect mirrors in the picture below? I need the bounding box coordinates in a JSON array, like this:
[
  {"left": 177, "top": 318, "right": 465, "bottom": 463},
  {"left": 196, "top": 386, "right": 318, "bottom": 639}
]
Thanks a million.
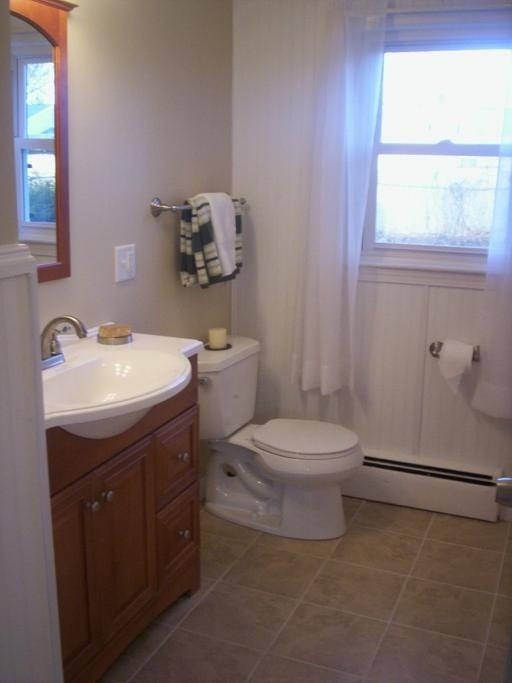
[{"left": 9, "top": 0, "right": 80, "bottom": 282}]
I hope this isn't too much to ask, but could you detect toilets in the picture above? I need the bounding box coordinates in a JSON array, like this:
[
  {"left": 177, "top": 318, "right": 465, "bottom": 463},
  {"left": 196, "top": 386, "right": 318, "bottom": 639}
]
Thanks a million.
[{"left": 199, "top": 333, "right": 363, "bottom": 541}]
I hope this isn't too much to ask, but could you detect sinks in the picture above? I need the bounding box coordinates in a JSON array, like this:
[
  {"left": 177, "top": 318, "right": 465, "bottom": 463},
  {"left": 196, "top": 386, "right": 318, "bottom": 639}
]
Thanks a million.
[{"left": 41, "top": 321, "right": 204, "bottom": 441}]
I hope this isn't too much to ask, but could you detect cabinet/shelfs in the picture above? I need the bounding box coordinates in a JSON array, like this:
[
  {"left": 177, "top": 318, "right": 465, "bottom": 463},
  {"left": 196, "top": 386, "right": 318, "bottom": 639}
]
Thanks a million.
[{"left": 44, "top": 354, "right": 200, "bottom": 683}]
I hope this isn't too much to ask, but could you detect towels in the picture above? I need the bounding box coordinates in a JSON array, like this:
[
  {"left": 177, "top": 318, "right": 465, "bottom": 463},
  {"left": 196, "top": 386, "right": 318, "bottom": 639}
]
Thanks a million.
[
  {"left": 179, "top": 196, "right": 242, "bottom": 290},
  {"left": 193, "top": 192, "right": 238, "bottom": 277}
]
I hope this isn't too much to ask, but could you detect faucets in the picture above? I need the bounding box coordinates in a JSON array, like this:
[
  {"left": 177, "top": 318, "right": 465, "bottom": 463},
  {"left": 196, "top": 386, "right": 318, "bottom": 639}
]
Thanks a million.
[{"left": 40, "top": 314, "right": 88, "bottom": 372}]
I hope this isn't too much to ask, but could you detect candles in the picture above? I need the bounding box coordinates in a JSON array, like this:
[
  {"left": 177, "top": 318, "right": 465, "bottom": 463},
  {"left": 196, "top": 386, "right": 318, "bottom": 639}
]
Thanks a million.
[{"left": 208, "top": 327, "right": 227, "bottom": 350}]
